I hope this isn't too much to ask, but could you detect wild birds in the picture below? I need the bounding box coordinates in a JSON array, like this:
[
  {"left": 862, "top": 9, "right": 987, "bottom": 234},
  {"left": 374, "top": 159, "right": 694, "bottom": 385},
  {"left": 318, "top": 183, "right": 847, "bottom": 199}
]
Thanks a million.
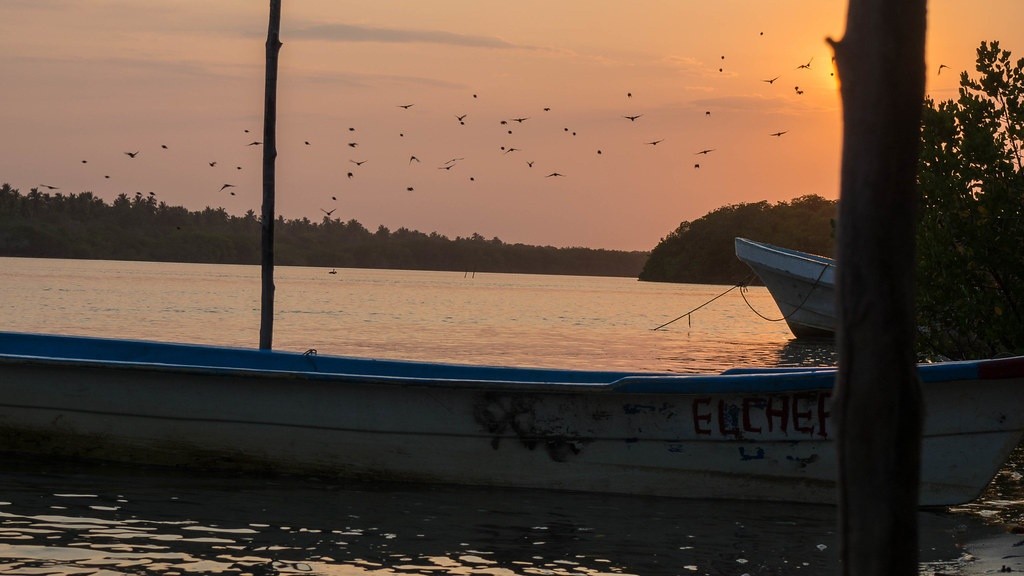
[
  {"left": 71, "top": 31, "right": 836, "bottom": 216},
  {"left": 937, "top": 65, "right": 952, "bottom": 76}
]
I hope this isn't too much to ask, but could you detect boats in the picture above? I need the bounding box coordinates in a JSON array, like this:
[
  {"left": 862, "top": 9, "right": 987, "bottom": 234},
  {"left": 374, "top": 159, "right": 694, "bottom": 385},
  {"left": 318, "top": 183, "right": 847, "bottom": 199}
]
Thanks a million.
[
  {"left": 1, "top": 325, "right": 1024, "bottom": 512},
  {"left": 732, "top": 236, "right": 837, "bottom": 339}
]
